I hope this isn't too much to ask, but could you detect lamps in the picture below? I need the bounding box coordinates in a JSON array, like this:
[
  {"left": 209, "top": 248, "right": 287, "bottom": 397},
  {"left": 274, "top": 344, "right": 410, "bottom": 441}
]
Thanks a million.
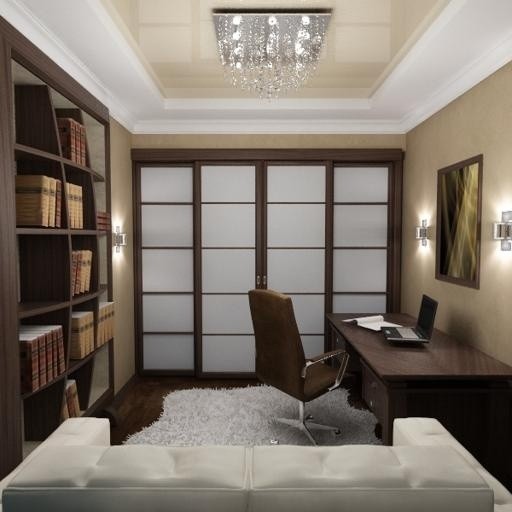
[
  {"left": 491, "top": 209, "right": 512, "bottom": 251},
  {"left": 116, "top": 223, "right": 126, "bottom": 254},
  {"left": 209, "top": 6, "right": 334, "bottom": 104},
  {"left": 414, "top": 219, "right": 432, "bottom": 248}
]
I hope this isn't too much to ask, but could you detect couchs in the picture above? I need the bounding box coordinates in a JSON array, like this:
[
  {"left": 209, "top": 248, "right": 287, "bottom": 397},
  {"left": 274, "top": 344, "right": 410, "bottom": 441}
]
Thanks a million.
[{"left": 1, "top": 413, "right": 511, "bottom": 512}]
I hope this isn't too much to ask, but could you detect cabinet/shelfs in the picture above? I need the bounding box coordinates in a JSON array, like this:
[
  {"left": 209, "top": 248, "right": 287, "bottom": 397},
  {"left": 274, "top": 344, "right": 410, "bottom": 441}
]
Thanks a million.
[{"left": 0, "top": 51, "right": 115, "bottom": 480}]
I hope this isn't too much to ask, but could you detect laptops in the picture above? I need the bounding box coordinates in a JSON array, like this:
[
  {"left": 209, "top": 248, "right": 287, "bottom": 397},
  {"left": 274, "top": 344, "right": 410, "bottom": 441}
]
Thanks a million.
[{"left": 381, "top": 294, "right": 438, "bottom": 343}]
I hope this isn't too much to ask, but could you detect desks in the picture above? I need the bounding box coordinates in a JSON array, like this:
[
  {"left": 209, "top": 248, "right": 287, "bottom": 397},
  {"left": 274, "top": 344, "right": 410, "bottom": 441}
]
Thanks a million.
[{"left": 326, "top": 310, "right": 511, "bottom": 491}]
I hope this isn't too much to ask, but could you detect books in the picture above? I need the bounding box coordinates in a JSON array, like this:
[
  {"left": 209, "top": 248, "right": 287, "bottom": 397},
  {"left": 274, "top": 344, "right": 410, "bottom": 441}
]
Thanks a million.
[
  {"left": 17, "top": 114, "right": 118, "bottom": 442},
  {"left": 342, "top": 314, "right": 403, "bottom": 332}
]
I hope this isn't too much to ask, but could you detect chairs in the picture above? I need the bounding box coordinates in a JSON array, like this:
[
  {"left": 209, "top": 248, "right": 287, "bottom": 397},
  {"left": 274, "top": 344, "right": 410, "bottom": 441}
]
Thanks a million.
[{"left": 245, "top": 286, "right": 354, "bottom": 445}]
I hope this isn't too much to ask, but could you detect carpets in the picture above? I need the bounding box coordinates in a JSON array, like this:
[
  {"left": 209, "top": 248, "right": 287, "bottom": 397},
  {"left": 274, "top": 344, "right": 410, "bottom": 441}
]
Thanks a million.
[{"left": 123, "top": 385, "right": 385, "bottom": 447}]
